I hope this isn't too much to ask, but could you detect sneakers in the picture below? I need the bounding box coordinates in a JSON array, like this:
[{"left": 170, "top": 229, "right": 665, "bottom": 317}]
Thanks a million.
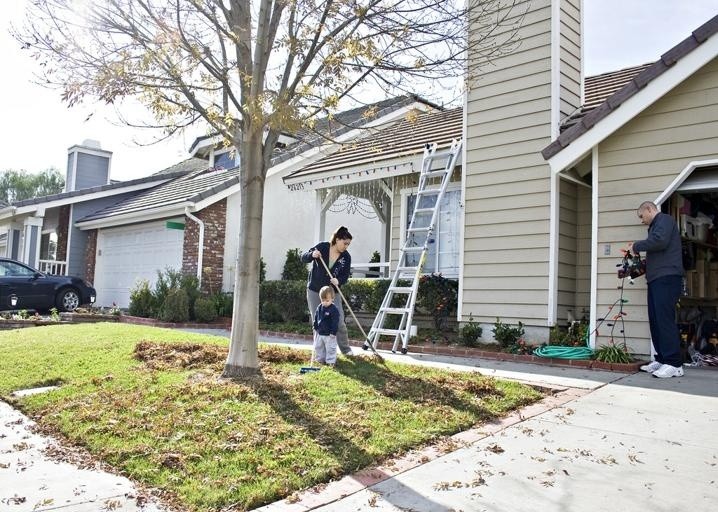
[
  {"left": 640, "top": 361, "right": 663, "bottom": 373},
  {"left": 319, "top": 361, "right": 336, "bottom": 369},
  {"left": 346, "top": 350, "right": 354, "bottom": 356},
  {"left": 651, "top": 363, "right": 684, "bottom": 377}
]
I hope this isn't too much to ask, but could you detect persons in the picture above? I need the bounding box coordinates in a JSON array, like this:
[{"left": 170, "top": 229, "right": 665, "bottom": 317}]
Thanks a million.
[
  {"left": 302, "top": 226, "right": 352, "bottom": 366},
  {"left": 629, "top": 201, "right": 684, "bottom": 378}
]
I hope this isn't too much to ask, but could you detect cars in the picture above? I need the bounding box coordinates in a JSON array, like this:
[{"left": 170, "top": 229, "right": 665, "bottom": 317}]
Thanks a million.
[{"left": 0, "top": 257, "right": 96, "bottom": 312}]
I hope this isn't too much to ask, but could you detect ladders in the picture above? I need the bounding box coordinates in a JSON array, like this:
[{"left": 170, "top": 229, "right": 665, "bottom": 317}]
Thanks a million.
[{"left": 362, "top": 137, "right": 462, "bottom": 353}]
